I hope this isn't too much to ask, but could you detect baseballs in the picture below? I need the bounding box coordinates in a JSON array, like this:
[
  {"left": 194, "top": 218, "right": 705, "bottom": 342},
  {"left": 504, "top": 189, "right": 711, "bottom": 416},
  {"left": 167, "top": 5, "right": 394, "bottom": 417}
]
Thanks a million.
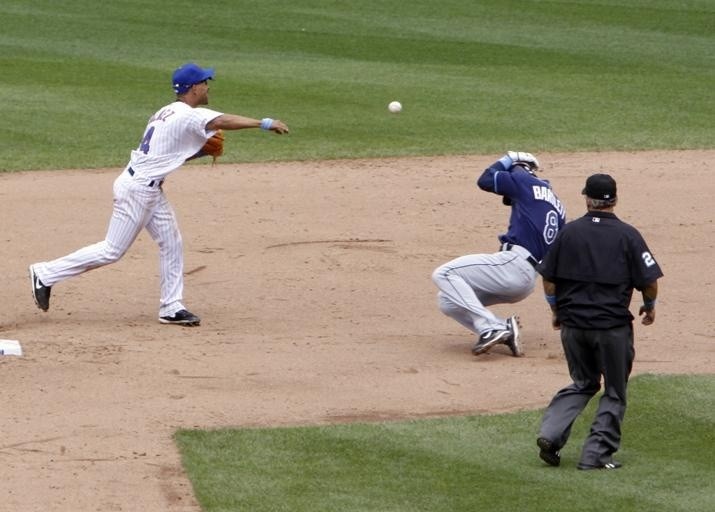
[{"left": 388, "top": 101, "right": 402, "bottom": 113}]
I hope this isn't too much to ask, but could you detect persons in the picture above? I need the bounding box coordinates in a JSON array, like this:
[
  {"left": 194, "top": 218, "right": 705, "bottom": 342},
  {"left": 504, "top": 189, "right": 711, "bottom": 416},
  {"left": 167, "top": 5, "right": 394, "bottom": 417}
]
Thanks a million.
[
  {"left": 29, "top": 64, "right": 288, "bottom": 325},
  {"left": 431, "top": 151, "right": 565, "bottom": 358},
  {"left": 534, "top": 171, "right": 663, "bottom": 470}
]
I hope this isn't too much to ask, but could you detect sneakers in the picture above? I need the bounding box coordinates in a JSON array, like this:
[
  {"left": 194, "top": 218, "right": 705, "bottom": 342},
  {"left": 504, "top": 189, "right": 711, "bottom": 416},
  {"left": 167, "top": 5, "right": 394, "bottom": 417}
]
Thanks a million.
[
  {"left": 577, "top": 457, "right": 624, "bottom": 472},
  {"left": 471, "top": 327, "right": 513, "bottom": 357},
  {"left": 535, "top": 437, "right": 561, "bottom": 468},
  {"left": 156, "top": 307, "right": 203, "bottom": 326},
  {"left": 504, "top": 314, "right": 526, "bottom": 358},
  {"left": 28, "top": 263, "right": 52, "bottom": 314}
]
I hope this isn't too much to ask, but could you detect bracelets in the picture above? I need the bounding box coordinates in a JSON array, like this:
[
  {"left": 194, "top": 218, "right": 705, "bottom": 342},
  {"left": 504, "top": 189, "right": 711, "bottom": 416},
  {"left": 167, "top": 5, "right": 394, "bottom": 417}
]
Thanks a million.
[
  {"left": 546, "top": 296, "right": 556, "bottom": 305},
  {"left": 644, "top": 299, "right": 657, "bottom": 307},
  {"left": 260, "top": 118, "right": 274, "bottom": 130}
]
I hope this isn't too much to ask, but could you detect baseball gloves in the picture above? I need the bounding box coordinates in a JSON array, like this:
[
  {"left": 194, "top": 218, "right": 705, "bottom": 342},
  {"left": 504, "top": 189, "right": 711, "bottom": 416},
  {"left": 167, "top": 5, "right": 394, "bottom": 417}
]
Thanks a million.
[{"left": 203, "top": 131, "right": 224, "bottom": 158}]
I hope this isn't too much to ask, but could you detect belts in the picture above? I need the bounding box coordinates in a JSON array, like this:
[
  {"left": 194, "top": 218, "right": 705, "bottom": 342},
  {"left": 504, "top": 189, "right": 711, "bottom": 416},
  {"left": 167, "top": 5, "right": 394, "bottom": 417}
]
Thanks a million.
[
  {"left": 126, "top": 165, "right": 165, "bottom": 189},
  {"left": 498, "top": 243, "right": 540, "bottom": 274}
]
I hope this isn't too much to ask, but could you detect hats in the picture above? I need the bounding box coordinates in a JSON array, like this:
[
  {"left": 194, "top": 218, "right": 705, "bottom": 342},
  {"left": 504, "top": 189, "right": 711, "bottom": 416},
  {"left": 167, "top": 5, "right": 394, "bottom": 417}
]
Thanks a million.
[
  {"left": 170, "top": 61, "right": 217, "bottom": 96},
  {"left": 580, "top": 173, "right": 619, "bottom": 202},
  {"left": 501, "top": 161, "right": 538, "bottom": 207}
]
